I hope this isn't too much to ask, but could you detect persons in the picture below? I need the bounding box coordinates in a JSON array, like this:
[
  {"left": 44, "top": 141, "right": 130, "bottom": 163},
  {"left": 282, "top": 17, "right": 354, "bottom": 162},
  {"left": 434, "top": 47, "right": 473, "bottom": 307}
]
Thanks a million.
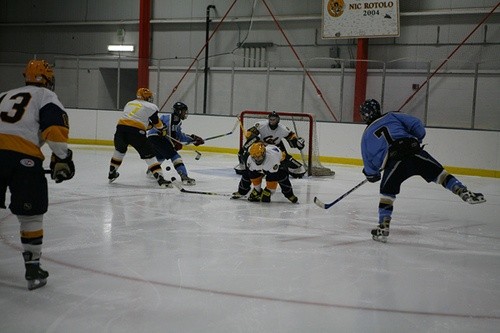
[
  {"left": 359, "top": 100, "right": 487, "bottom": 243},
  {"left": 232, "top": 111, "right": 305, "bottom": 203},
  {"left": 145, "top": 101, "right": 204, "bottom": 185},
  {"left": 245, "top": 143, "right": 282, "bottom": 203},
  {"left": 107, "top": 88, "right": 172, "bottom": 189},
  {"left": 0, "top": 61, "right": 76, "bottom": 289}
]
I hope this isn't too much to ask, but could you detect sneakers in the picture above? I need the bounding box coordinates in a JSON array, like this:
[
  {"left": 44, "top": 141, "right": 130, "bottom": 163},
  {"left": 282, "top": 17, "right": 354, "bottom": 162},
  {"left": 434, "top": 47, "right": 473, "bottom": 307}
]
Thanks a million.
[
  {"left": 108, "top": 166, "right": 120, "bottom": 184},
  {"left": 370, "top": 217, "right": 392, "bottom": 243},
  {"left": 181, "top": 176, "right": 197, "bottom": 185},
  {"left": 22, "top": 251, "right": 49, "bottom": 290},
  {"left": 286, "top": 193, "right": 299, "bottom": 204},
  {"left": 146, "top": 170, "right": 155, "bottom": 177},
  {"left": 155, "top": 175, "right": 173, "bottom": 188},
  {"left": 232, "top": 191, "right": 244, "bottom": 199},
  {"left": 455, "top": 187, "right": 487, "bottom": 205}
]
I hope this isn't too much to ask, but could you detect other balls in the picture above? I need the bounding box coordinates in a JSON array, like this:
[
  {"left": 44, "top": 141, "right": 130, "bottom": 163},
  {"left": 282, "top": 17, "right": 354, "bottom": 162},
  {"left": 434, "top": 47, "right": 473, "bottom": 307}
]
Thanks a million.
[{"left": 166, "top": 167, "right": 171, "bottom": 171}]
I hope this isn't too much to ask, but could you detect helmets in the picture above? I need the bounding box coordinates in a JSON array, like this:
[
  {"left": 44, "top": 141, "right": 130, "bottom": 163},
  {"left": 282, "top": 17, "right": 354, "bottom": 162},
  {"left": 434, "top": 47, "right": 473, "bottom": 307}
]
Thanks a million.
[
  {"left": 360, "top": 99, "right": 381, "bottom": 124},
  {"left": 268, "top": 111, "right": 280, "bottom": 127},
  {"left": 137, "top": 88, "right": 153, "bottom": 103},
  {"left": 23, "top": 59, "right": 56, "bottom": 92},
  {"left": 173, "top": 102, "right": 188, "bottom": 120},
  {"left": 249, "top": 143, "right": 267, "bottom": 165}
]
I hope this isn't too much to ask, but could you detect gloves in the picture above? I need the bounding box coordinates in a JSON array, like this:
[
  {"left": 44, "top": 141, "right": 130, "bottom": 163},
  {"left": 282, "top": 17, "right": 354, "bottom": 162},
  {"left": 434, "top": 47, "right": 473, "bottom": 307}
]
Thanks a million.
[
  {"left": 248, "top": 188, "right": 264, "bottom": 202},
  {"left": 146, "top": 121, "right": 154, "bottom": 130},
  {"left": 49, "top": 148, "right": 75, "bottom": 184},
  {"left": 158, "top": 122, "right": 169, "bottom": 136},
  {"left": 191, "top": 134, "right": 204, "bottom": 146},
  {"left": 261, "top": 188, "right": 272, "bottom": 203},
  {"left": 362, "top": 167, "right": 381, "bottom": 183}
]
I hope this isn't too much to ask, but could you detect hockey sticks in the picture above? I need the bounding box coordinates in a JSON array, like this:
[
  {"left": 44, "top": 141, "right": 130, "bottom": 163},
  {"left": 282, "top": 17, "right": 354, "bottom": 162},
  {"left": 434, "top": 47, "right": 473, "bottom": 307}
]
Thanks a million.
[
  {"left": 171, "top": 176, "right": 262, "bottom": 200},
  {"left": 236, "top": 114, "right": 249, "bottom": 140},
  {"left": 313, "top": 167, "right": 384, "bottom": 210},
  {"left": 166, "top": 135, "right": 202, "bottom": 161},
  {"left": 203, "top": 120, "right": 239, "bottom": 141},
  {"left": 43, "top": 169, "right": 66, "bottom": 175},
  {"left": 291, "top": 116, "right": 308, "bottom": 171}
]
